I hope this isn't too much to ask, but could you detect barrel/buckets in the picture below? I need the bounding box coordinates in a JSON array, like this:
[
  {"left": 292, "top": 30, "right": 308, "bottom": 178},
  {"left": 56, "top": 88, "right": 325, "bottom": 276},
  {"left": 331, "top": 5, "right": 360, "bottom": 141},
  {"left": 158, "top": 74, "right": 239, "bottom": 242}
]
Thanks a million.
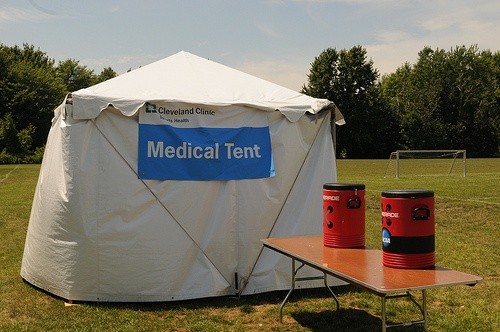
[
  {"left": 380, "top": 190, "right": 436, "bottom": 271},
  {"left": 322, "top": 182, "right": 365, "bottom": 249}
]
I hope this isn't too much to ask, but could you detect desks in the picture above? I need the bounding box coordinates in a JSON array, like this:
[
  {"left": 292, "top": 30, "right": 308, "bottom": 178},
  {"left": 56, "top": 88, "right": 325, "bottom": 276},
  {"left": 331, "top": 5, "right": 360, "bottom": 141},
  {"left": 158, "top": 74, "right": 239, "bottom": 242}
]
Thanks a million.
[{"left": 261, "top": 234, "right": 483, "bottom": 332}]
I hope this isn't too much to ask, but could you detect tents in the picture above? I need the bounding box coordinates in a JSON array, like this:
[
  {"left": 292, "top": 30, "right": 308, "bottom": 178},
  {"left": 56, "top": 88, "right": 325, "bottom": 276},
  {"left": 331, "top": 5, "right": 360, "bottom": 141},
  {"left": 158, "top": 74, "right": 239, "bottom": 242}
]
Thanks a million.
[{"left": 16, "top": 48, "right": 351, "bottom": 304}]
